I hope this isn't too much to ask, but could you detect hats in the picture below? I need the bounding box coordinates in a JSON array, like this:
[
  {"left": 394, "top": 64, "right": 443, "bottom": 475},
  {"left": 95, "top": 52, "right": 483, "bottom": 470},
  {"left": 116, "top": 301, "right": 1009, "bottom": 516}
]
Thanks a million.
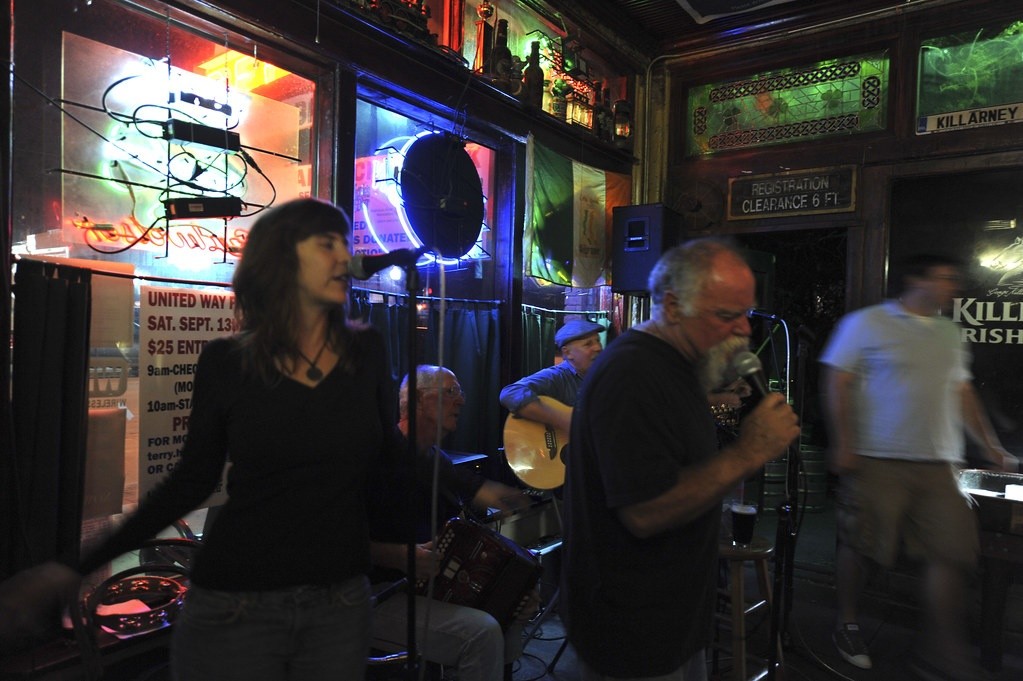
[{"left": 554, "top": 319, "right": 605, "bottom": 348}]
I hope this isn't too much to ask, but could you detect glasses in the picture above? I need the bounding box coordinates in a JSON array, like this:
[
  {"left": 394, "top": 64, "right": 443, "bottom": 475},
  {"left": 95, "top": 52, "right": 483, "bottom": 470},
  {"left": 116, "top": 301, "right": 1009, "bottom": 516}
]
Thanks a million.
[
  {"left": 925, "top": 275, "right": 967, "bottom": 286},
  {"left": 419, "top": 385, "right": 466, "bottom": 399}
]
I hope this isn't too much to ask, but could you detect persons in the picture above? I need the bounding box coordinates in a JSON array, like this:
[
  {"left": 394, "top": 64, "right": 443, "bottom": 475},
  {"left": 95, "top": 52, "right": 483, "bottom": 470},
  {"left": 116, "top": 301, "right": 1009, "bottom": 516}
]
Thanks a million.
[
  {"left": 814, "top": 248, "right": 1018, "bottom": 681},
  {"left": 69, "top": 198, "right": 528, "bottom": 681},
  {"left": 369, "top": 364, "right": 539, "bottom": 681},
  {"left": 499, "top": 319, "right": 605, "bottom": 607},
  {"left": 557, "top": 237, "right": 801, "bottom": 681}
]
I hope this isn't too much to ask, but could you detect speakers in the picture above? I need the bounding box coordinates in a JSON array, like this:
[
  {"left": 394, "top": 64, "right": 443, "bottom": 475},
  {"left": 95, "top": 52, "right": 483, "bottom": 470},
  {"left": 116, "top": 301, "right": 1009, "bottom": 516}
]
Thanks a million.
[{"left": 610, "top": 202, "right": 684, "bottom": 298}]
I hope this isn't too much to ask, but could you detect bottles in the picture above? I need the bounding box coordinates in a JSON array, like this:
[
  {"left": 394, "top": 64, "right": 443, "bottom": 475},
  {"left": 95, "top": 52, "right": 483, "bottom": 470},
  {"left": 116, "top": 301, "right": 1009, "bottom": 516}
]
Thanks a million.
[
  {"left": 489, "top": 19, "right": 511, "bottom": 96},
  {"left": 522, "top": 41, "right": 543, "bottom": 109},
  {"left": 602, "top": 88, "right": 614, "bottom": 142},
  {"left": 592, "top": 81, "right": 606, "bottom": 139}
]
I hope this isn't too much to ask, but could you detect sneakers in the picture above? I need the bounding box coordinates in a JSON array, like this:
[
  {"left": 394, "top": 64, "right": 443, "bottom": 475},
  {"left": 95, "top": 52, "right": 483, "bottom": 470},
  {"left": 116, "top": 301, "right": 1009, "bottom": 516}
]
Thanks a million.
[
  {"left": 831, "top": 622, "right": 872, "bottom": 669},
  {"left": 905, "top": 650, "right": 992, "bottom": 681}
]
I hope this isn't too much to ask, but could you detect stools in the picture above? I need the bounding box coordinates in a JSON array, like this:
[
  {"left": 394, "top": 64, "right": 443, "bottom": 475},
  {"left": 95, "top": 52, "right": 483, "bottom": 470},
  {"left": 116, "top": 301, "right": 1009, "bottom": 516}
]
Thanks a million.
[{"left": 719, "top": 536, "right": 784, "bottom": 681}]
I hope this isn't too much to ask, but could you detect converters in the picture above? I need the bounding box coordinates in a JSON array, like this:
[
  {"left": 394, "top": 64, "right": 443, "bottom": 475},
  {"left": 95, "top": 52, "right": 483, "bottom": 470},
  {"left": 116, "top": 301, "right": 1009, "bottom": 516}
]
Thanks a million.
[
  {"left": 164, "top": 119, "right": 240, "bottom": 155},
  {"left": 164, "top": 196, "right": 241, "bottom": 220}
]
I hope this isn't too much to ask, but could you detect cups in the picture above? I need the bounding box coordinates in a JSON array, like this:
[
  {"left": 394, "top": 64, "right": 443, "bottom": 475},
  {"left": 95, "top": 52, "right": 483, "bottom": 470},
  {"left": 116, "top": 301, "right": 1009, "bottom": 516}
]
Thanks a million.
[{"left": 730, "top": 500, "right": 757, "bottom": 550}]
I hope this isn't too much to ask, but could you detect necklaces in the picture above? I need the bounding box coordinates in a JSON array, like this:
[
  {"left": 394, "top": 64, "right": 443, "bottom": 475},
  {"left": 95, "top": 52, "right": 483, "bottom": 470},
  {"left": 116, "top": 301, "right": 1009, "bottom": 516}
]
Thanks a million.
[{"left": 296, "top": 340, "right": 329, "bottom": 381}]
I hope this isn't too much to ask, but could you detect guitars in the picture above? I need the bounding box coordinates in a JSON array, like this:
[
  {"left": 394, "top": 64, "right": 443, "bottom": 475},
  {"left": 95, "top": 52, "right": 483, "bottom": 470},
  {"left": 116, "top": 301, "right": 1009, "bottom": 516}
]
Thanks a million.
[{"left": 502, "top": 395, "right": 740, "bottom": 490}]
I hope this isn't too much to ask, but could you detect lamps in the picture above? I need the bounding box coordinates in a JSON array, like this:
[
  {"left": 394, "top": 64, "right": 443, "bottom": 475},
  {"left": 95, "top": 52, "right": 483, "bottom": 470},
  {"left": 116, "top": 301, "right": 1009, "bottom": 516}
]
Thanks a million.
[{"left": 374, "top": 120, "right": 491, "bottom": 269}]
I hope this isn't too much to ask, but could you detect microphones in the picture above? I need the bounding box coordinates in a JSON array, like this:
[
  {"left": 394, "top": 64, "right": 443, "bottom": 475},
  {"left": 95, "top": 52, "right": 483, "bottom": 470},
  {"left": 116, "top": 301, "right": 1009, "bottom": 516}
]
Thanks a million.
[
  {"left": 732, "top": 351, "right": 771, "bottom": 409},
  {"left": 348, "top": 246, "right": 429, "bottom": 280}
]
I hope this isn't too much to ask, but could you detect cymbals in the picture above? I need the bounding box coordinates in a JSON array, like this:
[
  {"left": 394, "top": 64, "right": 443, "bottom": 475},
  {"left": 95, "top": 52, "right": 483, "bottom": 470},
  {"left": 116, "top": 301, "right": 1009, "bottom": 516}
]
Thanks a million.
[{"left": 85, "top": 574, "right": 189, "bottom": 632}]
